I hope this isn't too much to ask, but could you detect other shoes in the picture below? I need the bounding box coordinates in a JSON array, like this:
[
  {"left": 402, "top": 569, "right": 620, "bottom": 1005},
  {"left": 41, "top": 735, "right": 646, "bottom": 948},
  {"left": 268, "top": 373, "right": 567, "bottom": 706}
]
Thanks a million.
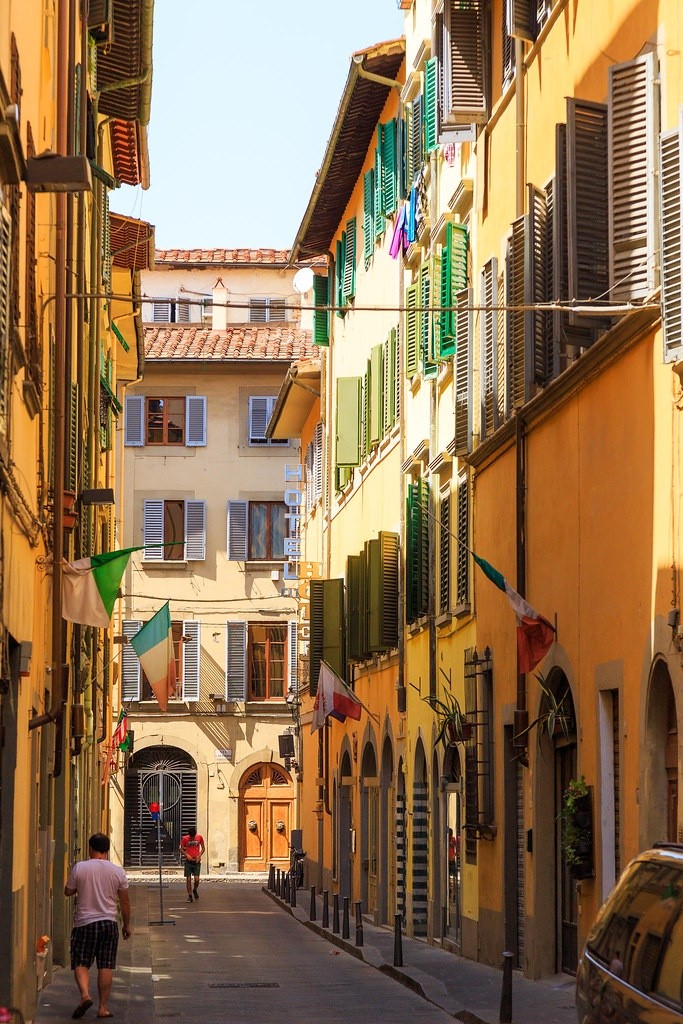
[
  {"left": 187, "top": 898, "right": 192, "bottom": 903},
  {"left": 193, "top": 889, "right": 199, "bottom": 898}
]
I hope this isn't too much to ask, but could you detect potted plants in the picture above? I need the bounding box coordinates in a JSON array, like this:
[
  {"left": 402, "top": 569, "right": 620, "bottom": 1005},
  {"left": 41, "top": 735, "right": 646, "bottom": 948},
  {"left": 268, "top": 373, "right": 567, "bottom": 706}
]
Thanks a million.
[
  {"left": 561, "top": 818, "right": 593, "bottom": 863},
  {"left": 512, "top": 667, "right": 573, "bottom": 765},
  {"left": 419, "top": 683, "right": 473, "bottom": 754}
]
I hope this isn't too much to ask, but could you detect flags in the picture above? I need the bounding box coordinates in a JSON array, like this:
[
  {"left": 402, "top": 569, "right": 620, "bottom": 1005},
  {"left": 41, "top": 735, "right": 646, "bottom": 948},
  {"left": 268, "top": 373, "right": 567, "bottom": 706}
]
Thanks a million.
[
  {"left": 112, "top": 709, "right": 130, "bottom": 752},
  {"left": 63, "top": 540, "right": 186, "bottom": 629},
  {"left": 311, "top": 659, "right": 362, "bottom": 734},
  {"left": 471, "top": 550, "right": 554, "bottom": 673},
  {"left": 131, "top": 602, "right": 177, "bottom": 712}
]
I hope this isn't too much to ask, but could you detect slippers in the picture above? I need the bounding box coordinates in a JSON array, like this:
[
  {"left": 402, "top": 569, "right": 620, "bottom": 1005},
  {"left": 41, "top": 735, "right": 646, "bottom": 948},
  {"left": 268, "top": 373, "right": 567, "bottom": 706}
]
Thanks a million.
[
  {"left": 97, "top": 1012, "right": 113, "bottom": 1018},
  {"left": 72, "top": 1000, "right": 93, "bottom": 1018}
]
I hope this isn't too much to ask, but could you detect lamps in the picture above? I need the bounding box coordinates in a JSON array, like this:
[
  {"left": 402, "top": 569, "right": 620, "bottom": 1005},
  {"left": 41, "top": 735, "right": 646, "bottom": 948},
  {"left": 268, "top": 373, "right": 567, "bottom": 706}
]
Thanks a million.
[{"left": 81, "top": 488, "right": 115, "bottom": 506}]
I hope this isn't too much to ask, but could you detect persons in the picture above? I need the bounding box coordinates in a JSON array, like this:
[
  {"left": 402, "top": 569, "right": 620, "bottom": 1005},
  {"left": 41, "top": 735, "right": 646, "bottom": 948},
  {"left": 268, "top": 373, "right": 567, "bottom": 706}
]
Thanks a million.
[
  {"left": 609, "top": 950, "right": 624, "bottom": 980},
  {"left": 178, "top": 827, "right": 205, "bottom": 902},
  {"left": 448, "top": 828, "right": 458, "bottom": 894},
  {"left": 64, "top": 832, "right": 132, "bottom": 1017}
]
